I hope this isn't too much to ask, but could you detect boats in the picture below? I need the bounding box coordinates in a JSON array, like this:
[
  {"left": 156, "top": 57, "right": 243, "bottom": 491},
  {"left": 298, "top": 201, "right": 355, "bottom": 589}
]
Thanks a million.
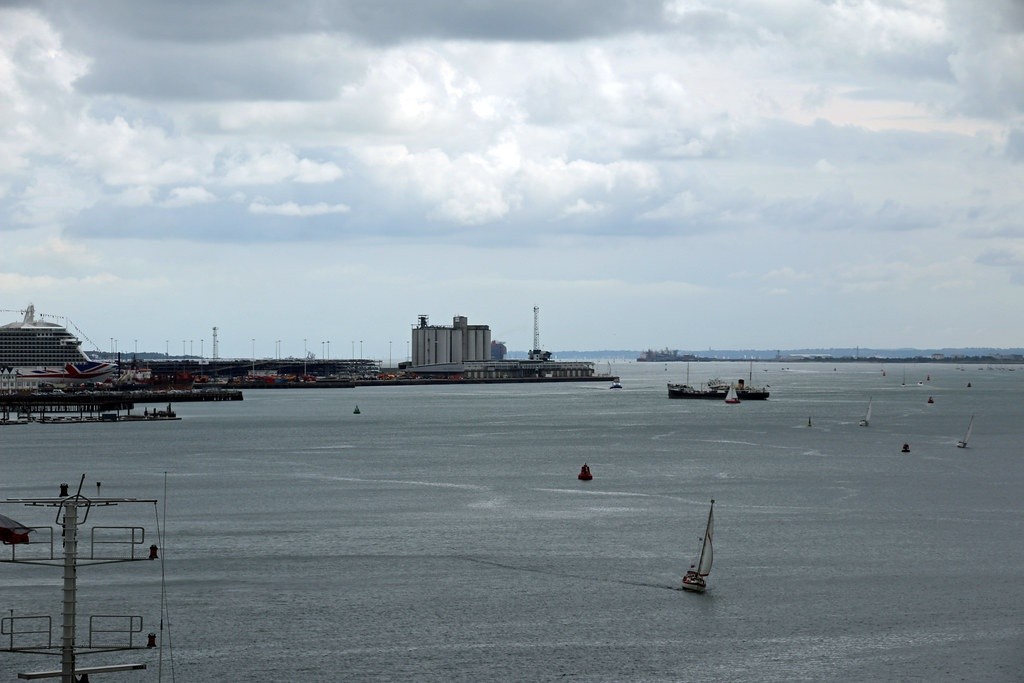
[
  {"left": 609, "top": 378, "right": 623, "bottom": 389},
  {"left": 577, "top": 462, "right": 593, "bottom": 480},
  {"left": 353, "top": 403, "right": 361, "bottom": 414},
  {"left": 667, "top": 357, "right": 770, "bottom": 400}
]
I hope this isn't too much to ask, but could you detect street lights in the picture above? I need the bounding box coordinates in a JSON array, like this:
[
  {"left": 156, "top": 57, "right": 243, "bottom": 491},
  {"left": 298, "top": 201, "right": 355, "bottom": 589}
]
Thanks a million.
[
  {"left": 252, "top": 339, "right": 254, "bottom": 379},
  {"left": 165, "top": 340, "right": 169, "bottom": 358},
  {"left": 327, "top": 341, "right": 330, "bottom": 363},
  {"left": 201, "top": 340, "right": 203, "bottom": 358},
  {"left": 275, "top": 340, "right": 281, "bottom": 360},
  {"left": 360, "top": 340, "right": 362, "bottom": 373},
  {"left": 406, "top": 341, "right": 409, "bottom": 365},
  {"left": 182, "top": 340, "right": 193, "bottom": 361},
  {"left": 389, "top": 341, "right": 392, "bottom": 374},
  {"left": 322, "top": 340, "right": 325, "bottom": 360},
  {"left": 111, "top": 337, "right": 118, "bottom": 363}
]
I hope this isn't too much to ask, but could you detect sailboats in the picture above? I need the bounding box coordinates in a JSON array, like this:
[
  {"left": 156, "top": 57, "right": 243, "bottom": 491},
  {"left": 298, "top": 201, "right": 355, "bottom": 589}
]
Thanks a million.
[
  {"left": 859, "top": 396, "right": 872, "bottom": 426},
  {"left": 957, "top": 414, "right": 974, "bottom": 449},
  {"left": 724, "top": 382, "right": 741, "bottom": 403},
  {"left": 682, "top": 500, "right": 716, "bottom": 592}
]
